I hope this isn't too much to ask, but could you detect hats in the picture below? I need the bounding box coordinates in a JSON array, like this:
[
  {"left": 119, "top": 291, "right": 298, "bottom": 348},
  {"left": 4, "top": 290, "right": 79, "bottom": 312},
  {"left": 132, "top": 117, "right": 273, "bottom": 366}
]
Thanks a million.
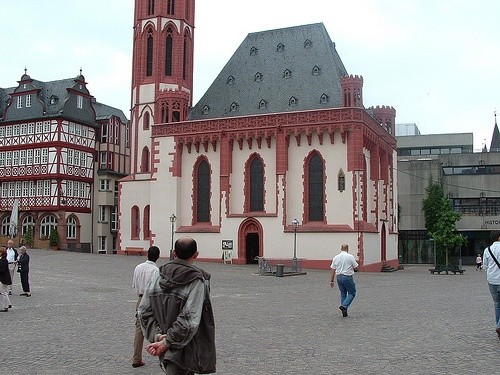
[{"left": 20, "top": 246, "right": 26, "bottom": 250}]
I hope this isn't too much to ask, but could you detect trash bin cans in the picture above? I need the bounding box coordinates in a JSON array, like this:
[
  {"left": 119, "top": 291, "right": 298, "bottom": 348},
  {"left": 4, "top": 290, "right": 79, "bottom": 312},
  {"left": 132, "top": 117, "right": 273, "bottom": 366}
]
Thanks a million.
[{"left": 276, "top": 264, "right": 284, "bottom": 276}]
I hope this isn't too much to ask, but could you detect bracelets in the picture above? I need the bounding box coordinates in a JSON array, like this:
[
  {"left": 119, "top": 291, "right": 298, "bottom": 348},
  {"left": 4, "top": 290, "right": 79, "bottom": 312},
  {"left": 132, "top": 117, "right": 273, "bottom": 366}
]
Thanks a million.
[{"left": 330, "top": 281, "right": 333, "bottom": 283}]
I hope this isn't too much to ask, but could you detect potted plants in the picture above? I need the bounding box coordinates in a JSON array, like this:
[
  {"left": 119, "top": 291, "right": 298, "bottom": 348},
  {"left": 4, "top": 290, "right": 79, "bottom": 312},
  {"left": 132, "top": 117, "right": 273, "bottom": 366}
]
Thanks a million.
[
  {"left": 48, "top": 226, "right": 58, "bottom": 251},
  {"left": 21, "top": 224, "right": 35, "bottom": 249}
]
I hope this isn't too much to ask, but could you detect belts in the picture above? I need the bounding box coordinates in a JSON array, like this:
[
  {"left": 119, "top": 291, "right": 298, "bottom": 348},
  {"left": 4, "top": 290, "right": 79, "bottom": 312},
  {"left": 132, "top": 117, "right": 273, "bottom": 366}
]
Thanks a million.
[
  {"left": 8, "top": 262, "right": 13, "bottom": 264},
  {"left": 139, "top": 294, "right": 143, "bottom": 297}
]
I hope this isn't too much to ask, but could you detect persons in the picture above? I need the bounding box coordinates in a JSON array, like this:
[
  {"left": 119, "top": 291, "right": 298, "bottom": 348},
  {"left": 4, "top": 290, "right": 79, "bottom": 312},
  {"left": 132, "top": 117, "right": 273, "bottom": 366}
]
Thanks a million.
[
  {"left": 483, "top": 230, "right": 500, "bottom": 339},
  {"left": 0, "top": 247, "right": 12, "bottom": 311},
  {"left": 330, "top": 244, "right": 359, "bottom": 317},
  {"left": 132, "top": 246, "right": 161, "bottom": 368},
  {"left": 476, "top": 254, "right": 482, "bottom": 271},
  {"left": 5, "top": 240, "right": 18, "bottom": 295},
  {"left": 138, "top": 237, "right": 216, "bottom": 375},
  {"left": 13, "top": 246, "right": 31, "bottom": 297}
]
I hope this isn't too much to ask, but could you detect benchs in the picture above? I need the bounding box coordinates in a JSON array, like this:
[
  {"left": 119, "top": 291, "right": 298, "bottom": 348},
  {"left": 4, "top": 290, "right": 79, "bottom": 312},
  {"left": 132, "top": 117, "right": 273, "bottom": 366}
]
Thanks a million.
[
  {"left": 123, "top": 247, "right": 144, "bottom": 256},
  {"left": 429, "top": 266, "right": 466, "bottom": 274}
]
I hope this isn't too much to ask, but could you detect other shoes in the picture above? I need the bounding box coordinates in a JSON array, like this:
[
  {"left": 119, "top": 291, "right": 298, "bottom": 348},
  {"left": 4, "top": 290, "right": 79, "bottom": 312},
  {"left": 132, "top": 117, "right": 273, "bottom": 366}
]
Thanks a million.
[
  {"left": 339, "top": 306, "right": 347, "bottom": 317},
  {"left": 26, "top": 293, "right": 31, "bottom": 297},
  {"left": 495, "top": 328, "right": 500, "bottom": 337},
  {"left": 0, "top": 293, "right": 2, "bottom": 295},
  {"left": 8, "top": 305, "right": 12, "bottom": 308},
  {"left": 19, "top": 293, "right": 27, "bottom": 296},
  {"left": 132, "top": 363, "right": 145, "bottom": 367},
  {"left": 8, "top": 292, "right": 12, "bottom": 295},
  {"left": 0, "top": 308, "right": 8, "bottom": 312}
]
